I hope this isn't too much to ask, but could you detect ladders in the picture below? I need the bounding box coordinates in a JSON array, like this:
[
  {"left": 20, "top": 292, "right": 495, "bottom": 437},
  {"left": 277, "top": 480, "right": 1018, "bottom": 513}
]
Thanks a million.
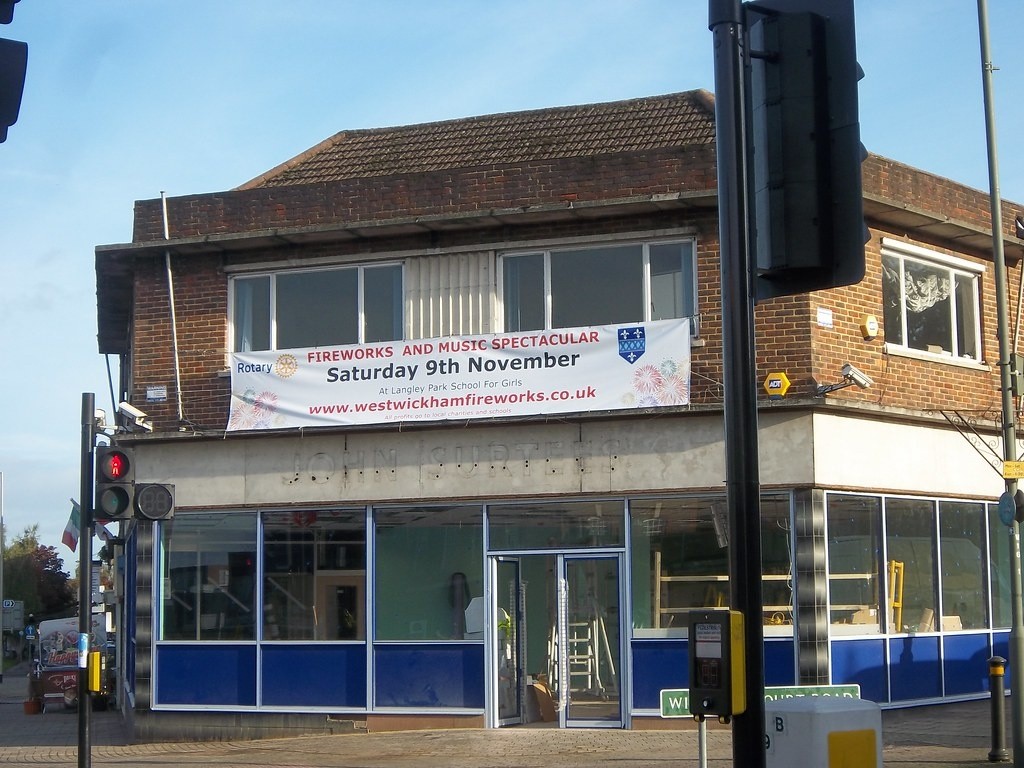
[{"left": 545, "top": 538, "right": 619, "bottom": 703}]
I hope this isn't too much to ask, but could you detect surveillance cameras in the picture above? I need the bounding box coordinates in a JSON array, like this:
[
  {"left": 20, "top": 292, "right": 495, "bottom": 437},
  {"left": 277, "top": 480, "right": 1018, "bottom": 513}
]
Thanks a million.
[
  {"left": 841, "top": 363, "right": 875, "bottom": 390},
  {"left": 119, "top": 401, "right": 148, "bottom": 425}
]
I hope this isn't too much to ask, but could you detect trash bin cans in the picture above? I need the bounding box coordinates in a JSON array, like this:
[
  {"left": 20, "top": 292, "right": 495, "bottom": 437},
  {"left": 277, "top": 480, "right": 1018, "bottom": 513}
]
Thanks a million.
[{"left": 765, "top": 696, "right": 884, "bottom": 768}]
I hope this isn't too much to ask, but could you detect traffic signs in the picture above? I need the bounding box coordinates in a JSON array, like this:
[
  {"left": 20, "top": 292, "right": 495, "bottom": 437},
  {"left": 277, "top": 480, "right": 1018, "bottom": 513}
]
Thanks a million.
[{"left": 3, "top": 599, "right": 16, "bottom": 608}]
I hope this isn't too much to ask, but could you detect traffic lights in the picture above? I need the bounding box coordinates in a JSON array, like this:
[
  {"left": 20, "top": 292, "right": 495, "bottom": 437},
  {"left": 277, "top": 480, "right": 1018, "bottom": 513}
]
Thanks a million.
[
  {"left": 91, "top": 445, "right": 178, "bottom": 524},
  {"left": 685, "top": 606, "right": 748, "bottom": 724},
  {"left": 87, "top": 652, "right": 105, "bottom": 693},
  {"left": 0, "top": 0, "right": 31, "bottom": 143}
]
